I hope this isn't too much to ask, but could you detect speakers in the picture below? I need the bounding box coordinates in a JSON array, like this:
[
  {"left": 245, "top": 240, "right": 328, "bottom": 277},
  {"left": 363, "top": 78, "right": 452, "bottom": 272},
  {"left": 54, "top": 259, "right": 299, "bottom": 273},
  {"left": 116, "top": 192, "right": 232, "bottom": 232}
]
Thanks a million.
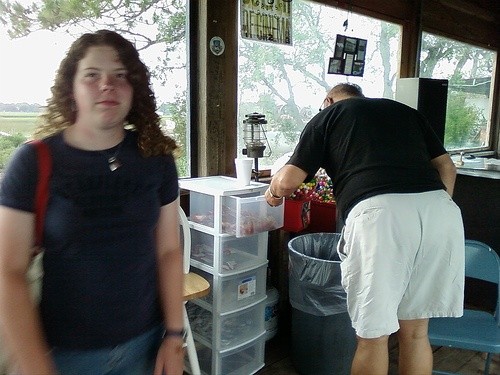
[{"left": 396, "top": 77, "right": 448, "bottom": 148}]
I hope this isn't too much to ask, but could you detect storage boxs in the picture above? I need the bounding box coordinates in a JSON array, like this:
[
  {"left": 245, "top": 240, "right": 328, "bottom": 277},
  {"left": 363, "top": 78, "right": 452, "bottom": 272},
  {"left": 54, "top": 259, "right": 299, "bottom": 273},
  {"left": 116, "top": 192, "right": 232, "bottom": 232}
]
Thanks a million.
[{"left": 185, "top": 183, "right": 284, "bottom": 375}]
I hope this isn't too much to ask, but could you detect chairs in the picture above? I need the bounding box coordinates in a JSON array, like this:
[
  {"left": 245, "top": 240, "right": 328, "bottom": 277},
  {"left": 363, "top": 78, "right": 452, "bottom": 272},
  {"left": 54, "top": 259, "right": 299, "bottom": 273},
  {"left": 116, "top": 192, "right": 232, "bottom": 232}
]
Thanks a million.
[
  {"left": 176, "top": 203, "right": 210, "bottom": 375},
  {"left": 427, "top": 239, "right": 500, "bottom": 375}
]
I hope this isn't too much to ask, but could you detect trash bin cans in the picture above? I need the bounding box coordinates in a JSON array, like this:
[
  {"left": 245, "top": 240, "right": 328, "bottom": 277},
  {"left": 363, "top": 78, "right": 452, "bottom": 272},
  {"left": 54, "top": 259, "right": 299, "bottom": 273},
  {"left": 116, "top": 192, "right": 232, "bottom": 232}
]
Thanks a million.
[{"left": 287, "top": 232, "right": 357, "bottom": 375}]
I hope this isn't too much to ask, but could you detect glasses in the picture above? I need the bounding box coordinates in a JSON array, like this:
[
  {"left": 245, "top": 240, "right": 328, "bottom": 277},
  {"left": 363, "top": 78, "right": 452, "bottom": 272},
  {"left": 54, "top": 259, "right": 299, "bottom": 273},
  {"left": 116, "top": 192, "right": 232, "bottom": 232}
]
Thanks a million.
[{"left": 318, "top": 98, "right": 328, "bottom": 112}]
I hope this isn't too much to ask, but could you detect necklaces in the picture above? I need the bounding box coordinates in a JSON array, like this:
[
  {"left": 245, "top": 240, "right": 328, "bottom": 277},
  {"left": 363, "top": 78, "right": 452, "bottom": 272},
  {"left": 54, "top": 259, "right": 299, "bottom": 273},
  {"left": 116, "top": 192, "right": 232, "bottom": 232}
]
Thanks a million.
[{"left": 101, "top": 144, "right": 121, "bottom": 171}]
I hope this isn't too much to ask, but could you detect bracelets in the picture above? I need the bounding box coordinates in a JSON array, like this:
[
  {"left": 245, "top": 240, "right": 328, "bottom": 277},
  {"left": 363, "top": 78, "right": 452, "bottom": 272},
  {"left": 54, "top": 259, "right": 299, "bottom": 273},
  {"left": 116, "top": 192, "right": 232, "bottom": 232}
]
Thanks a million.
[
  {"left": 268, "top": 187, "right": 283, "bottom": 200},
  {"left": 162, "top": 328, "right": 186, "bottom": 338}
]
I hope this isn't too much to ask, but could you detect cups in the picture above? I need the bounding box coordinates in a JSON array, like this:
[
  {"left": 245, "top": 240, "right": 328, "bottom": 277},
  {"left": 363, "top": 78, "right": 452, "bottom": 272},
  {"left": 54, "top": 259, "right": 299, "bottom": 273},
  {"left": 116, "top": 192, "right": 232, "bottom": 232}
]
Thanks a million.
[{"left": 235, "top": 158, "right": 253, "bottom": 186}]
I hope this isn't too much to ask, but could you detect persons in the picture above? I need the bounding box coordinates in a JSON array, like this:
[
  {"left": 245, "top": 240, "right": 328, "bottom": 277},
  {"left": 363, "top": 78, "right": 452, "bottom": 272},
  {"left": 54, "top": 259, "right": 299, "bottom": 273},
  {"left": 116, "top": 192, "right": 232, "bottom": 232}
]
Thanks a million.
[
  {"left": 0, "top": 29, "right": 186, "bottom": 375},
  {"left": 264, "top": 84, "right": 465, "bottom": 375}
]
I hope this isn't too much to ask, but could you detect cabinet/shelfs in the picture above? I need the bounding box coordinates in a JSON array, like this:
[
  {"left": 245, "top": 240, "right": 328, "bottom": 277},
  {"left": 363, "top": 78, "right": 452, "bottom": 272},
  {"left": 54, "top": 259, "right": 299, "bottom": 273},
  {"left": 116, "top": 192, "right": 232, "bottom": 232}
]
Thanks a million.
[{"left": 177, "top": 175, "right": 285, "bottom": 375}]
